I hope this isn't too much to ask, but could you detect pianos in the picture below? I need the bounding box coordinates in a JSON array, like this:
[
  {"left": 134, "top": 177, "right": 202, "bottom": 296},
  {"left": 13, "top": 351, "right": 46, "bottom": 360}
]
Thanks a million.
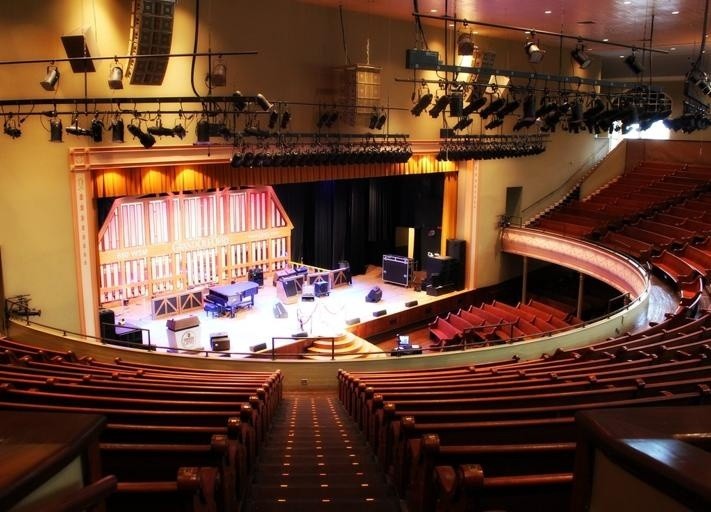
[{"left": 204, "top": 280, "right": 259, "bottom": 318}]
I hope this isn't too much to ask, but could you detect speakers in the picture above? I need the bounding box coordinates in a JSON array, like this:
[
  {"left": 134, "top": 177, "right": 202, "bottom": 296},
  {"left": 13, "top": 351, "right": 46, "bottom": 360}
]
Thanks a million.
[
  {"left": 345, "top": 317, "right": 361, "bottom": 326},
  {"left": 406, "top": 300, "right": 418, "bottom": 306},
  {"left": 372, "top": 309, "right": 386, "bottom": 317},
  {"left": 124, "top": 0, "right": 174, "bottom": 86},
  {"left": 60, "top": 25, "right": 99, "bottom": 73},
  {"left": 461, "top": 50, "right": 497, "bottom": 102},
  {"left": 249, "top": 342, "right": 266, "bottom": 352},
  {"left": 406, "top": 49, "right": 438, "bottom": 70},
  {"left": 292, "top": 332, "right": 308, "bottom": 339}
]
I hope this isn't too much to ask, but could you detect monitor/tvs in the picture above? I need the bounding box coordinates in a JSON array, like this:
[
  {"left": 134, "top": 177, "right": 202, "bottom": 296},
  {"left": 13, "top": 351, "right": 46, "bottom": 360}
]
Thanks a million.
[{"left": 399, "top": 335, "right": 409, "bottom": 346}]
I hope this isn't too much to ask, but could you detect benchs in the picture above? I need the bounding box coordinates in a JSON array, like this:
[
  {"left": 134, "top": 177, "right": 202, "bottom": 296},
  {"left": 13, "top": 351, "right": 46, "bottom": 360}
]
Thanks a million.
[
  {"left": 526, "top": 176, "right": 711, "bottom": 303},
  {"left": 0, "top": 340, "right": 282, "bottom": 512},
  {"left": 337, "top": 303, "right": 711, "bottom": 511},
  {"left": 426, "top": 301, "right": 584, "bottom": 355}
]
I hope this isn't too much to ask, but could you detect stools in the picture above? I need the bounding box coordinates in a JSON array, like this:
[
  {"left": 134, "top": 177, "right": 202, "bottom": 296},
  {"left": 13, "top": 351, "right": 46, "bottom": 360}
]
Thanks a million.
[{"left": 204, "top": 304, "right": 218, "bottom": 317}]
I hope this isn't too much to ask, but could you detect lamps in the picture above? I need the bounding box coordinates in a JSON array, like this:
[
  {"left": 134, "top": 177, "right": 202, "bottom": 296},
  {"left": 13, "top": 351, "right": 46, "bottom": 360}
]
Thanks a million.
[{"left": 0, "top": 0, "right": 711, "bottom": 170}]
